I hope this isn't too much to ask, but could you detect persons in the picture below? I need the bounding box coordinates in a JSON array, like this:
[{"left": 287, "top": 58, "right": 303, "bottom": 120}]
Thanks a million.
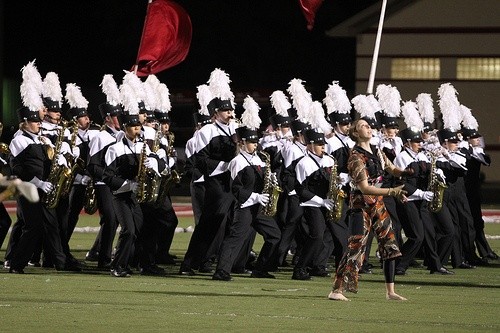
[
  {"left": 328, "top": 119, "right": 409, "bottom": 301},
  {"left": 351, "top": 83, "right": 500, "bottom": 274},
  {"left": 0, "top": 58, "right": 179, "bottom": 278},
  {"left": 180, "top": 68, "right": 357, "bottom": 280}
]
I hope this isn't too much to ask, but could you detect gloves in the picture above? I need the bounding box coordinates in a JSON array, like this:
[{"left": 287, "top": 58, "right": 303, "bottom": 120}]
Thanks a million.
[
  {"left": 143, "top": 157, "right": 158, "bottom": 174},
  {"left": 156, "top": 148, "right": 166, "bottom": 159},
  {"left": 257, "top": 194, "right": 270, "bottom": 207},
  {"left": 387, "top": 184, "right": 407, "bottom": 203},
  {"left": 122, "top": 180, "right": 140, "bottom": 194},
  {"left": 323, "top": 199, "right": 335, "bottom": 211},
  {"left": 72, "top": 147, "right": 80, "bottom": 157},
  {"left": 337, "top": 173, "right": 350, "bottom": 186},
  {"left": 79, "top": 173, "right": 91, "bottom": 185},
  {"left": 270, "top": 172, "right": 278, "bottom": 186},
  {"left": 418, "top": 146, "right": 484, "bottom": 202},
  {"left": 29, "top": 176, "right": 55, "bottom": 195},
  {"left": 55, "top": 154, "right": 68, "bottom": 169}
]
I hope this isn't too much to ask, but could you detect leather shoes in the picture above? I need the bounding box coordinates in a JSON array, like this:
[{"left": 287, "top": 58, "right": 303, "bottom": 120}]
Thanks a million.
[
  {"left": 178, "top": 249, "right": 330, "bottom": 281},
  {"left": 380, "top": 247, "right": 499, "bottom": 275},
  {"left": 359, "top": 259, "right": 374, "bottom": 274},
  {"left": 84, "top": 250, "right": 178, "bottom": 276},
  {"left": 0, "top": 255, "right": 87, "bottom": 274}
]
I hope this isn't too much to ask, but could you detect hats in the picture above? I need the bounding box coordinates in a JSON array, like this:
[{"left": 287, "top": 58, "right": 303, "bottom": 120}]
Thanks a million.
[
  {"left": 16, "top": 59, "right": 89, "bottom": 123},
  {"left": 235, "top": 95, "right": 263, "bottom": 143},
  {"left": 270, "top": 78, "right": 400, "bottom": 146},
  {"left": 192, "top": 68, "right": 235, "bottom": 126},
  {"left": 98, "top": 70, "right": 171, "bottom": 128},
  {"left": 398, "top": 83, "right": 483, "bottom": 145}
]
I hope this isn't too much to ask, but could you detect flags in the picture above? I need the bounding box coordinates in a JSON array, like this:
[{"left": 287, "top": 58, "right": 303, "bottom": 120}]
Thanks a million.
[
  {"left": 299, "top": 0, "right": 318, "bottom": 30},
  {"left": 130, "top": 0, "right": 192, "bottom": 76}
]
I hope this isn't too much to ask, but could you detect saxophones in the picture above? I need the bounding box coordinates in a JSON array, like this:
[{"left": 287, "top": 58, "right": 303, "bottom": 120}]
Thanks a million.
[
  {"left": 254, "top": 149, "right": 284, "bottom": 217},
  {"left": 44, "top": 114, "right": 85, "bottom": 199},
  {"left": 82, "top": 121, "right": 102, "bottom": 217},
  {"left": 230, "top": 118, "right": 244, "bottom": 155},
  {"left": 137, "top": 133, "right": 162, "bottom": 209},
  {"left": 167, "top": 131, "right": 181, "bottom": 194},
  {"left": 153, "top": 127, "right": 170, "bottom": 202},
  {"left": 322, "top": 151, "right": 345, "bottom": 223},
  {"left": 67, "top": 119, "right": 79, "bottom": 153},
  {"left": 38, "top": 127, "right": 71, "bottom": 209},
  {"left": 420, "top": 148, "right": 449, "bottom": 213},
  {"left": 0, "top": 124, "right": 14, "bottom": 160}
]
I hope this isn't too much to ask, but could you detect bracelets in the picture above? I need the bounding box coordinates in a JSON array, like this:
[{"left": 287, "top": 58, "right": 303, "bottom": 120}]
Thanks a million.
[{"left": 387, "top": 188, "right": 390, "bottom": 196}]
